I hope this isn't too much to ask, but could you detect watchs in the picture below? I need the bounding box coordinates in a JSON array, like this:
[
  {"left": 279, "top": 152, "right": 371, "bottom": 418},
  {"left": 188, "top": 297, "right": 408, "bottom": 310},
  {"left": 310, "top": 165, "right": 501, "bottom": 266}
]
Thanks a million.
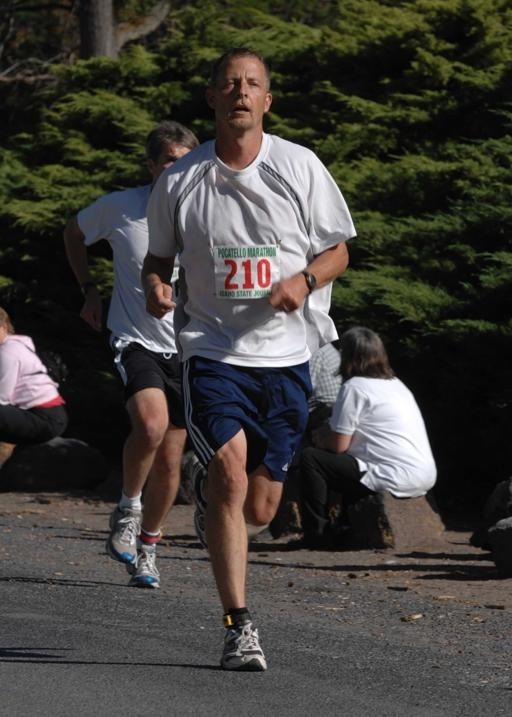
[{"left": 300, "top": 268, "right": 317, "bottom": 294}]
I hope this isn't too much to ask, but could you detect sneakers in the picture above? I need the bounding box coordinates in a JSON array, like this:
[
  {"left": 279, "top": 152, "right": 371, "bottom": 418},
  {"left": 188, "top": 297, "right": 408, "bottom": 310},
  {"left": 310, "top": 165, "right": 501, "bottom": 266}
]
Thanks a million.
[
  {"left": 189, "top": 461, "right": 209, "bottom": 549},
  {"left": 221, "top": 622, "right": 268, "bottom": 671},
  {"left": 285, "top": 533, "right": 326, "bottom": 551},
  {"left": 105, "top": 505, "right": 144, "bottom": 565},
  {"left": 127, "top": 538, "right": 161, "bottom": 589}
]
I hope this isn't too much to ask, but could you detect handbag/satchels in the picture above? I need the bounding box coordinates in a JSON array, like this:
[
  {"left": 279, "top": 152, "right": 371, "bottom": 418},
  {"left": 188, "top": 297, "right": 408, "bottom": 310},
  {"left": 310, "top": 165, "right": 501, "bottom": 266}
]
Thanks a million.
[
  {"left": 47, "top": 371, "right": 73, "bottom": 411},
  {"left": 306, "top": 400, "right": 332, "bottom": 432}
]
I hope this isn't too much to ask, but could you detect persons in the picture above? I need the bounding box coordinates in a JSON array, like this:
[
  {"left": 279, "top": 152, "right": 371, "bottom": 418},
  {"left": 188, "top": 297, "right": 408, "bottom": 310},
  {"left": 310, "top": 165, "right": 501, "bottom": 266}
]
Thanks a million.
[
  {"left": 286, "top": 323, "right": 438, "bottom": 550},
  {"left": 1, "top": 307, "right": 72, "bottom": 472},
  {"left": 139, "top": 44, "right": 358, "bottom": 670},
  {"left": 60, "top": 116, "right": 201, "bottom": 589},
  {"left": 253, "top": 338, "right": 343, "bottom": 544}
]
